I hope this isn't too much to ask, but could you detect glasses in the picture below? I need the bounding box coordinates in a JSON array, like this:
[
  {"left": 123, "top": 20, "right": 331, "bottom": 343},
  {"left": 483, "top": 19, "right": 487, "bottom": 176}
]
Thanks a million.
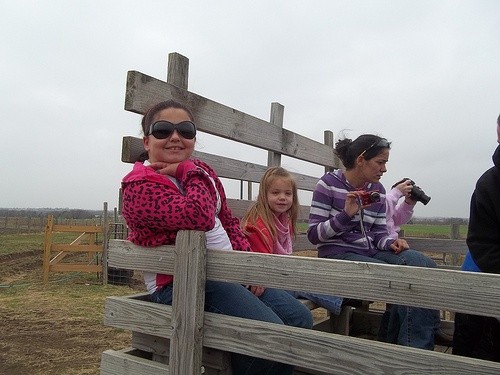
[
  {"left": 145, "top": 120, "right": 197, "bottom": 139},
  {"left": 360, "top": 138, "right": 392, "bottom": 156}
]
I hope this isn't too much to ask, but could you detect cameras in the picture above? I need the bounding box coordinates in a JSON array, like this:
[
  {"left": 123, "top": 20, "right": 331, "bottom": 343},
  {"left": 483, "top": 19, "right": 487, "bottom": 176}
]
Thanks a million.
[
  {"left": 359, "top": 190, "right": 381, "bottom": 208},
  {"left": 392, "top": 178, "right": 431, "bottom": 206}
]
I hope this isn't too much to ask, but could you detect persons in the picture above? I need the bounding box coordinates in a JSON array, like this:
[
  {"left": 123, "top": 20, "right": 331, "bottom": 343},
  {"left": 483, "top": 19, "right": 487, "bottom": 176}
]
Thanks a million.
[
  {"left": 121, "top": 100, "right": 313, "bottom": 375},
  {"left": 384, "top": 180, "right": 417, "bottom": 238},
  {"left": 243, "top": 166, "right": 375, "bottom": 315},
  {"left": 452, "top": 116, "right": 500, "bottom": 364},
  {"left": 306, "top": 135, "right": 440, "bottom": 352}
]
